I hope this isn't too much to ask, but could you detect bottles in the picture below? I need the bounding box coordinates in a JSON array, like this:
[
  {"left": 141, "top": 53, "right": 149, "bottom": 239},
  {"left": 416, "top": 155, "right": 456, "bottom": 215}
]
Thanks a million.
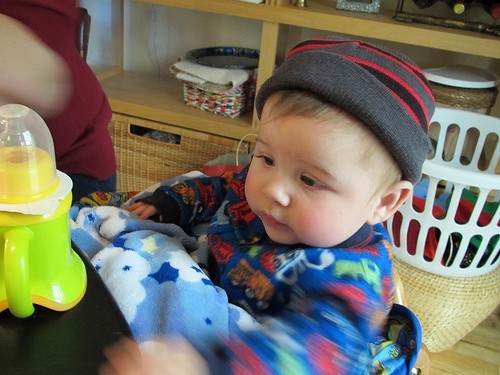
[{"left": 0, "top": 104, "right": 88, "bottom": 318}]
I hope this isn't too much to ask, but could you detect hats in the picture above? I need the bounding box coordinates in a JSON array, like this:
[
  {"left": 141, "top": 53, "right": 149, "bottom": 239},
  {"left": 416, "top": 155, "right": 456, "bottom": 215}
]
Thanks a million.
[{"left": 255, "top": 36, "right": 436, "bottom": 186}]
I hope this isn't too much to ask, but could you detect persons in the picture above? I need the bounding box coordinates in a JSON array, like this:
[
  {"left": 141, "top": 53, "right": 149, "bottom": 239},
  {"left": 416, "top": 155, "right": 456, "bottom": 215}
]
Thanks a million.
[
  {"left": 0, "top": 0, "right": 117, "bottom": 206},
  {"left": 98, "top": 36, "right": 436, "bottom": 375}
]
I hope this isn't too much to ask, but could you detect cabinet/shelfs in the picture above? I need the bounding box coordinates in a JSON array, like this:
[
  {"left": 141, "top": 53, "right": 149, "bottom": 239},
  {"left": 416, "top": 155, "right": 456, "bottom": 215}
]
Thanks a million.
[{"left": 76, "top": 0, "right": 500, "bottom": 194}]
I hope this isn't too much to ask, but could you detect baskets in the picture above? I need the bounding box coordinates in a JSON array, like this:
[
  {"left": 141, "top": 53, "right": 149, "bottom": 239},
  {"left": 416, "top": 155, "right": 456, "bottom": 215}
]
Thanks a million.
[{"left": 394, "top": 255, "right": 500, "bottom": 353}]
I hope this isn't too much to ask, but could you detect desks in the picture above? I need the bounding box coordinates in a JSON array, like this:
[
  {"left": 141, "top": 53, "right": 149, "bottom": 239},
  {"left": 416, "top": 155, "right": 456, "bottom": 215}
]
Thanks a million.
[{"left": 1, "top": 238, "right": 143, "bottom": 375}]
